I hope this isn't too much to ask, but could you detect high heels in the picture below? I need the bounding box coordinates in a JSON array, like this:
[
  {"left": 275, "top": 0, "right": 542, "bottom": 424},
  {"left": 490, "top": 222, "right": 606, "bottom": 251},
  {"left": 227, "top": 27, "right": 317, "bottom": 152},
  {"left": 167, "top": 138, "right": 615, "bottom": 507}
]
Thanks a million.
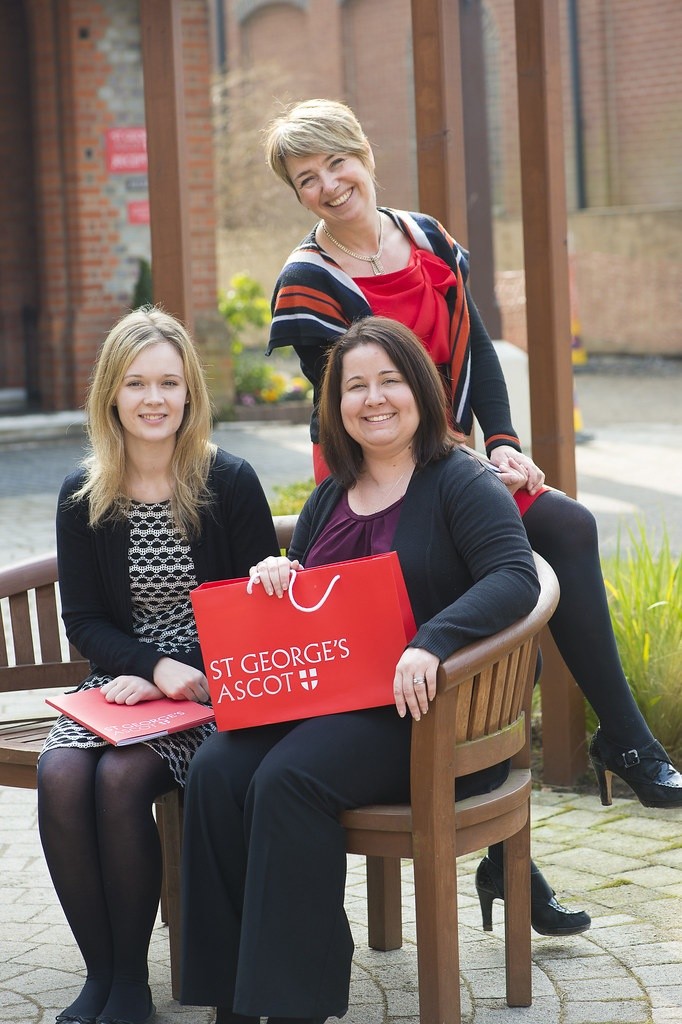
[
  {"left": 590, "top": 726, "right": 682, "bottom": 808},
  {"left": 475, "top": 854, "right": 590, "bottom": 935}
]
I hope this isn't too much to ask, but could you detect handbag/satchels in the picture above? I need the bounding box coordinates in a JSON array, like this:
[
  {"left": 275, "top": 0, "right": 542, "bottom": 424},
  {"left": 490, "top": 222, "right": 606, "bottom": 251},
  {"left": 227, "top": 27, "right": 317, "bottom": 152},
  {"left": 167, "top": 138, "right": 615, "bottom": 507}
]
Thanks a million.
[{"left": 189, "top": 551, "right": 419, "bottom": 733}]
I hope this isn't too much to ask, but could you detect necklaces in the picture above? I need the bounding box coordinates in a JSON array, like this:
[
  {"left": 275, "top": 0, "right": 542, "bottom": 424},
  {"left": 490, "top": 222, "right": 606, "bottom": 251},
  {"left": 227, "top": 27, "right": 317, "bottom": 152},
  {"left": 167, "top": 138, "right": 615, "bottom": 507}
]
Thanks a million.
[
  {"left": 322, "top": 211, "right": 386, "bottom": 275},
  {"left": 362, "top": 456, "right": 412, "bottom": 515}
]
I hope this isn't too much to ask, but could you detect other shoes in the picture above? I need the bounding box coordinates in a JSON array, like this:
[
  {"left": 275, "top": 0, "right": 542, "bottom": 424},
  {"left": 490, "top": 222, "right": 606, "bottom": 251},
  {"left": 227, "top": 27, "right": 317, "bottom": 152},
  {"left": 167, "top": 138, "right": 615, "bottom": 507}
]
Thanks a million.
[
  {"left": 96, "top": 984, "right": 156, "bottom": 1023},
  {"left": 55, "top": 1009, "right": 93, "bottom": 1023}
]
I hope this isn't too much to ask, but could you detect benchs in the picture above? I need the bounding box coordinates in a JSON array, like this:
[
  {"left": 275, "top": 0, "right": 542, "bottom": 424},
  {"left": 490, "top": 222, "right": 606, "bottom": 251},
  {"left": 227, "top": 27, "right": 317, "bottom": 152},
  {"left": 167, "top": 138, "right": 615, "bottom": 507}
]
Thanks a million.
[{"left": 0, "top": 514, "right": 561, "bottom": 1024}]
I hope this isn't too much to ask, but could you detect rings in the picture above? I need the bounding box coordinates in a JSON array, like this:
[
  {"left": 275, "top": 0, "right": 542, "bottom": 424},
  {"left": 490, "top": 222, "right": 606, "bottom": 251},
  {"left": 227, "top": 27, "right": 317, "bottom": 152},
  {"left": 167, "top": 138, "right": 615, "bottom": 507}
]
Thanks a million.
[{"left": 413, "top": 677, "right": 425, "bottom": 685}]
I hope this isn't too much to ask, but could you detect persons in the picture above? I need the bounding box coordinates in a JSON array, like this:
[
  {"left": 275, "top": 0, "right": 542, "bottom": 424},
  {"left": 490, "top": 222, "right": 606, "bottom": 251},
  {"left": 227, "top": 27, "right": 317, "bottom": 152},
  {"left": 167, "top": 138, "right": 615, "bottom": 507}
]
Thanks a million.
[
  {"left": 259, "top": 96, "right": 682, "bottom": 936},
  {"left": 33, "top": 307, "right": 284, "bottom": 1024},
  {"left": 166, "top": 317, "right": 542, "bottom": 1024}
]
